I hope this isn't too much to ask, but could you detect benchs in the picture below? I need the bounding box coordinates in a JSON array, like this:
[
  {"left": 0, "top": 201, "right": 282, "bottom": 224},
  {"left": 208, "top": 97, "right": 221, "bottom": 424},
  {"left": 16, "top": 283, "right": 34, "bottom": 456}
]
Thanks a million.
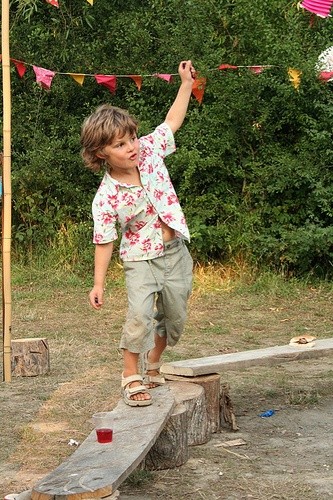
[
  {"left": 160, "top": 337, "right": 333, "bottom": 441},
  {"left": 31, "top": 388, "right": 189, "bottom": 500}
]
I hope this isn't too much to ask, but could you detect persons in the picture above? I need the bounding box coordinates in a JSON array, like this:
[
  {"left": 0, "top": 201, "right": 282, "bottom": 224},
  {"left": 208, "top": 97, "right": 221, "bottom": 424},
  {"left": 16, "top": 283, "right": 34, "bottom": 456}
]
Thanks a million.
[{"left": 81, "top": 60, "right": 195, "bottom": 407}]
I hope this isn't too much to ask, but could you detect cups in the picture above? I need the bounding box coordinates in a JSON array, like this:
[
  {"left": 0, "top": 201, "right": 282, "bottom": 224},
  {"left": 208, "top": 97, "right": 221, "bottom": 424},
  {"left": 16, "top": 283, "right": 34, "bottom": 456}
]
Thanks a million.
[{"left": 92, "top": 412, "right": 114, "bottom": 443}]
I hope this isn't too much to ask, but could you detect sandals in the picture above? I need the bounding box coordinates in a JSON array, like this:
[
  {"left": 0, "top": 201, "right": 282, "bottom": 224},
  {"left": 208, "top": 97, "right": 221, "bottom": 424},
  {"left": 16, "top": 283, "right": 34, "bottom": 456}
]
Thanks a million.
[
  {"left": 141, "top": 348, "right": 165, "bottom": 389},
  {"left": 120, "top": 370, "right": 154, "bottom": 406}
]
